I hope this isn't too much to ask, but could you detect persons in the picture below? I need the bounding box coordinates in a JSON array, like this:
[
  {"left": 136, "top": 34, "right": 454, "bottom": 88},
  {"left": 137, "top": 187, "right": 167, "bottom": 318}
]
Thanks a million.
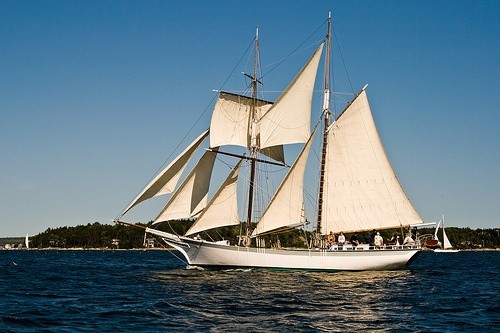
[
  {"left": 388, "top": 226, "right": 420, "bottom": 244},
  {"left": 370, "top": 229, "right": 383, "bottom": 247},
  {"left": 294, "top": 233, "right": 306, "bottom": 246},
  {"left": 307, "top": 230, "right": 316, "bottom": 247},
  {"left": 327, "top": 231, "right": 361, "bottom": 249},
  {"left": 243, "top": 231, "right": 254, "bottom": 248}
]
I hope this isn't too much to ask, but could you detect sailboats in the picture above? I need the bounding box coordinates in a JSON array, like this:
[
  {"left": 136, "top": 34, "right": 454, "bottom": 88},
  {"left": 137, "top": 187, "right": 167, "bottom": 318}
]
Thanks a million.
[
  {"left": 110, "top": 14, "right": 440, "bottom": 271},
  {"left": 430, "top": 213, "right": 462, "bottom": 254}
]
